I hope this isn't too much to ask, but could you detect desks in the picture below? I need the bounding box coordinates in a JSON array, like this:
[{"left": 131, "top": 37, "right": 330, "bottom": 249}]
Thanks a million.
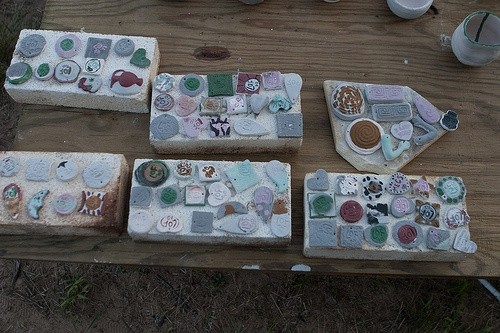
[{"left": 0, "top": 0, "right": 500, "bottom": 302}]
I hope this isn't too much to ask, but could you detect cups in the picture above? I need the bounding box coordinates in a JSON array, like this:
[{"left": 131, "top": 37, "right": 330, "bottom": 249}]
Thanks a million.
[
  {"left": 386, "top": 0, "right": 434, "bottom": 20},
  {"left": 451, "top": 12, "right": 499, "bottom": 68}
]
